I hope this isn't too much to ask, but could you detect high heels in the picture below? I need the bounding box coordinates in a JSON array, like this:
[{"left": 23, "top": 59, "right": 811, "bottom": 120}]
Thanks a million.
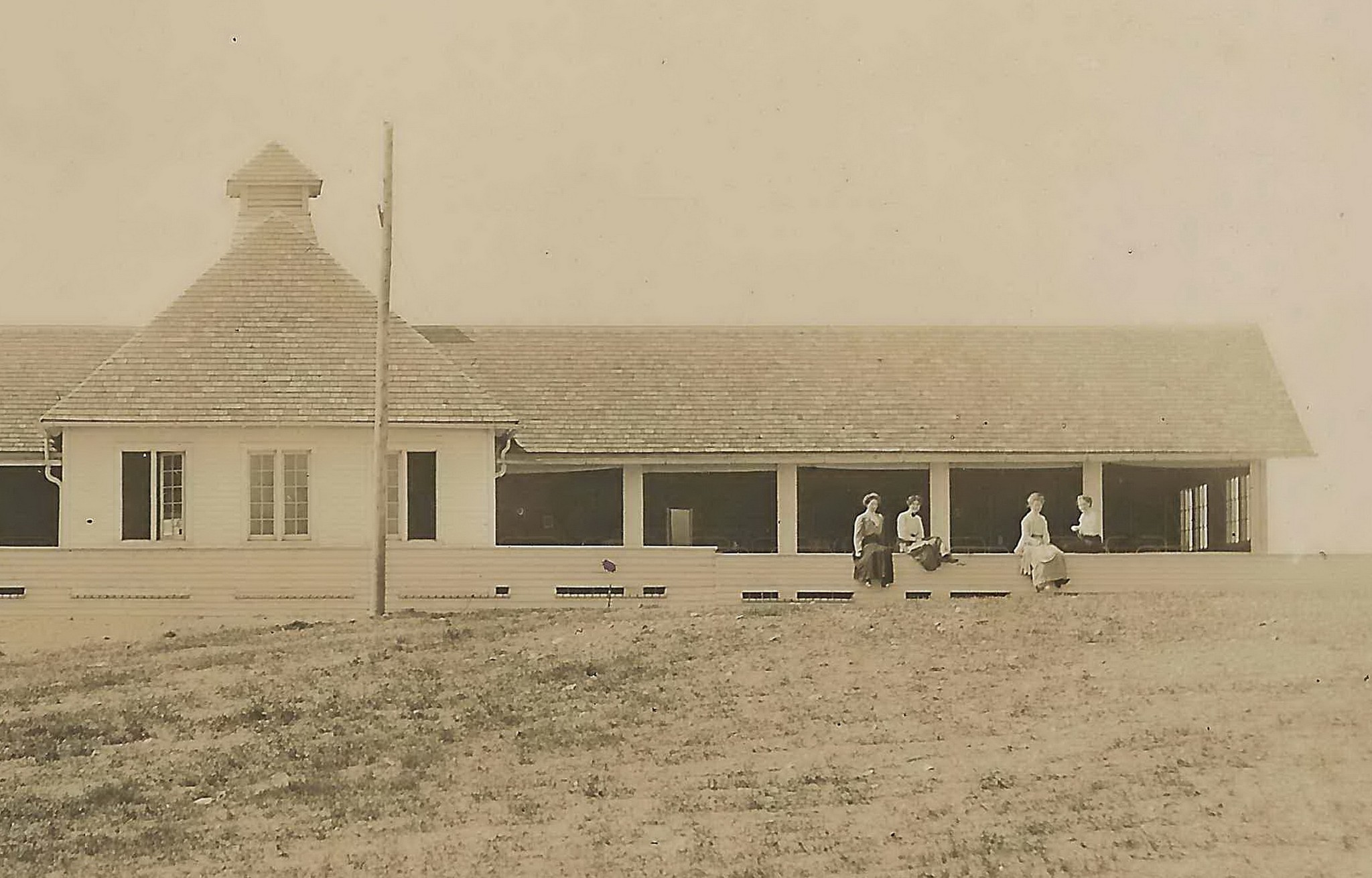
[{"left": 1054, "top": 578, "right": 1071, "bottom": 588}]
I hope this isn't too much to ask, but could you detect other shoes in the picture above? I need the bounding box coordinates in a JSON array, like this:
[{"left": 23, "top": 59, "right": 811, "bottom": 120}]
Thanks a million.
[
  {"left": 940, "top": 555, "right": 959, "bottom": 564},
  {"left": 861, "top": 577, "right": 873, "bottom": 587},
  {"left": 880, "top": 577, "right": 890, "bottom": 587}
]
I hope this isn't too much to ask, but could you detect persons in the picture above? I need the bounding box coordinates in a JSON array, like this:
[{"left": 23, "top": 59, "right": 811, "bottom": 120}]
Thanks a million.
[
  {"left": 1013, "top": 492, "right": 1072, "bottom": 591},
  {"left": 897, "top": 494, "right": 968, "bottom": 573},
  {"left": 851, "top": 491, "right": 894, "bottom": 589},
  {"left": 1070, "top": 495, "right": 1102, "bottom": 554}
]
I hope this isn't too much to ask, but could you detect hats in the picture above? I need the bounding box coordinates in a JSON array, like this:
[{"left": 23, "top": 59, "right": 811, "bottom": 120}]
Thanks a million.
[{"left": 862, "top": 492, "right": 881, "bottom": 508}]
